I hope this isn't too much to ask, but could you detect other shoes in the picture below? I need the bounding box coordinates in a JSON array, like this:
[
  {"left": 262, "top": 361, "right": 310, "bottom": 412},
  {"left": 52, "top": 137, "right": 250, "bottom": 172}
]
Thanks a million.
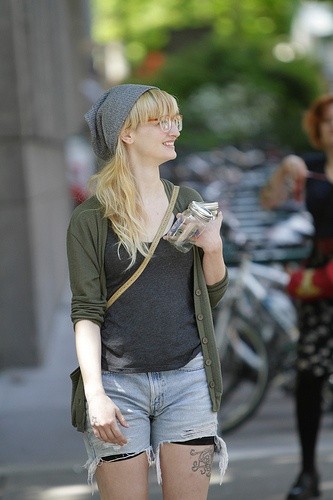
[{"left": 286, "top": 469, "right": 320, "bottom": 500}]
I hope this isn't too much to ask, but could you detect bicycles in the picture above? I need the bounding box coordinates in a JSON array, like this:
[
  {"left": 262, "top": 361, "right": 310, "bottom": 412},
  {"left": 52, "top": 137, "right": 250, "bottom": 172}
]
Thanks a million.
[{"left": 197, "top": 229, "right": 311, "bottom": 433}]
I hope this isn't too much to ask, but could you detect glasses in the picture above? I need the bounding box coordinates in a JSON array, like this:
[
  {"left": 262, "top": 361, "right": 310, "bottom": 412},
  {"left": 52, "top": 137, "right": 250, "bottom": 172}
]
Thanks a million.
[{"left": 154, "top": 115, "right": 183, "bottom": 133}]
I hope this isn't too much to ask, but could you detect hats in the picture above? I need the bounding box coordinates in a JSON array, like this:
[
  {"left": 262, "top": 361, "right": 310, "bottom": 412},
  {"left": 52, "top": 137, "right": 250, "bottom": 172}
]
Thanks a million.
[{"left": 83, "top": 83, "right": 160, "bottom": 162}]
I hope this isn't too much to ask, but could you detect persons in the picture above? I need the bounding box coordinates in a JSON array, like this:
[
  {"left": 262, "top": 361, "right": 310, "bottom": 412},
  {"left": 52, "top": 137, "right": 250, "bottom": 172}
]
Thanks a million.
[
  {"left": 67, "top": 84, "right": 229, "bottom": 500},
  {"left": 259, "top": 92, "right": 333, "bottom": 500}
]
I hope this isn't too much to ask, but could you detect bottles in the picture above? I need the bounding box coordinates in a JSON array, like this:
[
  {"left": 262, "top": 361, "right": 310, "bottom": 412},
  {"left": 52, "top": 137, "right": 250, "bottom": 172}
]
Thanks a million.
[{"left": 166, "top": 201, "right": 219, "bottom": 253}]
